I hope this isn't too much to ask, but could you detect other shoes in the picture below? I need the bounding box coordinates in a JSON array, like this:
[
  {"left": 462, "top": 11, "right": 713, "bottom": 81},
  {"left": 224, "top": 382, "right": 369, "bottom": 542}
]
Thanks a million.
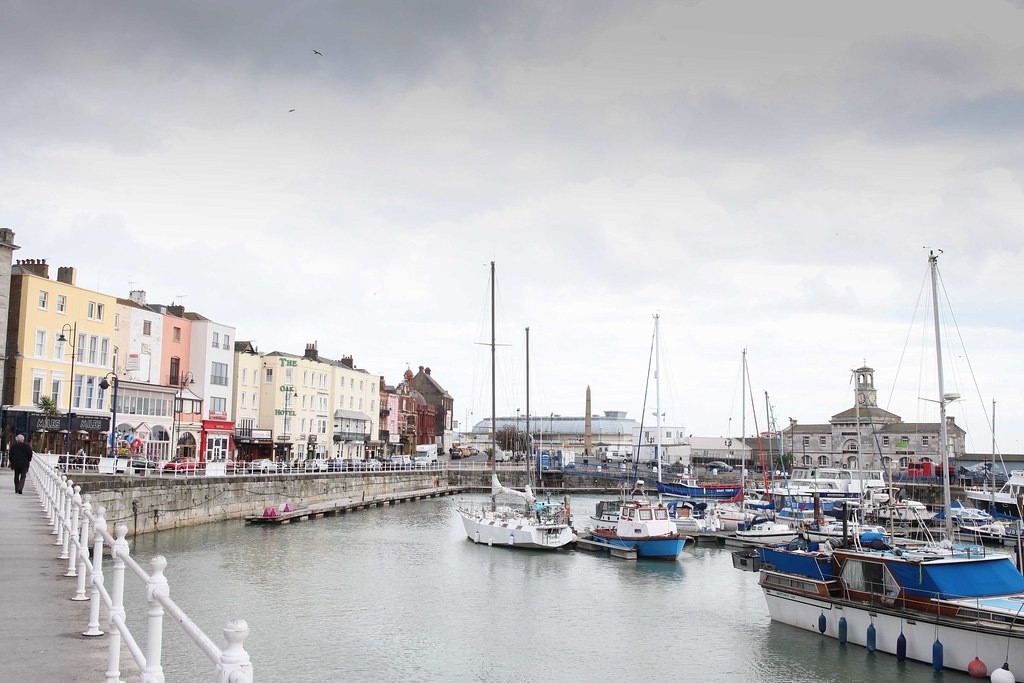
[{"left": 15, "top": 488, "right": 22, "bottom": 494}]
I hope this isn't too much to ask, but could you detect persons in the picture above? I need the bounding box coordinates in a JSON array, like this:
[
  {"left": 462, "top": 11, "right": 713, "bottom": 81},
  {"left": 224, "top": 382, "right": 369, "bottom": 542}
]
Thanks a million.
[
  {"left": 9, "top": 434, "right": 32, "bottom": 494},
  {"left": 276, "top": 455, "right": 284, "bottom": 462},
  {"left": 76, "top": 447, "right": 84, "bottom": 470}
]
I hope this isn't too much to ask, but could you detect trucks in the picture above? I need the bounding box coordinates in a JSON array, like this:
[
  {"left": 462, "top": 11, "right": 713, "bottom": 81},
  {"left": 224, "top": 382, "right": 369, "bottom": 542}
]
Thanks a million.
[{"left": 413, "top": 444, "right": 437, "bottom": 467}]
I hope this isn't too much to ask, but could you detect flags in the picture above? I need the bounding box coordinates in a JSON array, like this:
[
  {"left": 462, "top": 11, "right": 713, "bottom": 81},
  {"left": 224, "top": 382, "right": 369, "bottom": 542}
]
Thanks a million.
[
  {"left": 801, "top": 503, "right": 806, "bottom": 510},
  {"left": 819, "top": 518, "right": 824, "bottom": 526}
]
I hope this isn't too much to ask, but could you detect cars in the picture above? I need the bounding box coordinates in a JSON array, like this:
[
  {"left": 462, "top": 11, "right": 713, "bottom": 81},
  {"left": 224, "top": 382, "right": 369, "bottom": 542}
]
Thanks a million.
[
  {"left": 165, "top": 456, "right": 198, "bottom": 472},
  {"left": 305, "top": 458, "right": 383, "bottom": 472},
  {"left": 247, "top": 458, "right": 276, "bottom": 473}
]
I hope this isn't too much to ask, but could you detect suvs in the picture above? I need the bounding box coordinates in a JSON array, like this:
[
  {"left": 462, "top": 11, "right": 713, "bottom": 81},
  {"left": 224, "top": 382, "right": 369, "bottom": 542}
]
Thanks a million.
[{"left": 109, "top": 452, "right": 156, "bottom": 473}]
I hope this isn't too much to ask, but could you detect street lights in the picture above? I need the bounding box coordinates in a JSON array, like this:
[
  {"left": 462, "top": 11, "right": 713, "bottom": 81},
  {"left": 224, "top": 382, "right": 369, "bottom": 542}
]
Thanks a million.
[
  {"left": 178, "top": 370, "right": 194, "bottom": 446},
  {"left": 98, "top": 371, "right": 118, "bottom": 458},
  {"left": 57, "top": 321, "right": 76, "bottom": 463}
]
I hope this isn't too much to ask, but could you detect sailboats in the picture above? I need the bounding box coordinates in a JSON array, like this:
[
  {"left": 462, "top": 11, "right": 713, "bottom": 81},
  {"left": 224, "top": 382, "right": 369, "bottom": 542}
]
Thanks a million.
[
  {"left": 589, "top": 246, "right": 1024, "bottom": 683},
  {"left": 455, "top": 259, "right": 578, "bottom": 550}
]
